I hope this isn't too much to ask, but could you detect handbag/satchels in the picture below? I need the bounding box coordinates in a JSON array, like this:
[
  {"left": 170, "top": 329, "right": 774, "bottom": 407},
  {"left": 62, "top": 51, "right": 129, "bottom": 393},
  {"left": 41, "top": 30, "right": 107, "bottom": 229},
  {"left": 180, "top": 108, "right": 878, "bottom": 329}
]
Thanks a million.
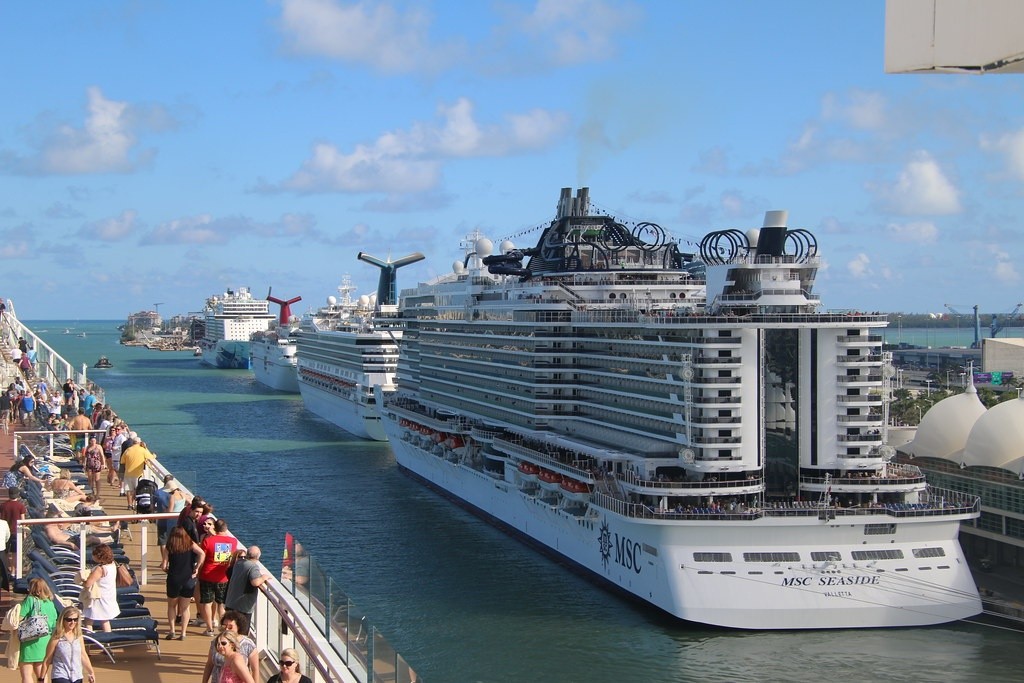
[
  {"left": 113, "top": 559, "right": 135, "bottom": 588},
  {"left": 17, "top": 597, "right": 49, "bottom": 643}
]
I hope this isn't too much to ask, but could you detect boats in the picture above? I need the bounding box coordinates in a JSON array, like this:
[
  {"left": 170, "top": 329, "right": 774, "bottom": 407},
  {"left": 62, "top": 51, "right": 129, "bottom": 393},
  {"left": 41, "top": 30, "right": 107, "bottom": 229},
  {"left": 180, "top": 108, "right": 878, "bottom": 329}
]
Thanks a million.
[
  {"left": 247, "top": 285, "right": 303, "bottom": 394},
  {"left": 194, "top": 348, "right": 202, "bottom": 356},
  {"left": 372, "top": 182, "right": 986, "bottom": 630},
  {"left": 198, "top": 286, "right": 276, "bottom": 370},
  {"left": 293, "top": 245, "right": 425, "bottom": 442},
  {"left": 94, "top": 357, "right": 114, "bottom": 368}
]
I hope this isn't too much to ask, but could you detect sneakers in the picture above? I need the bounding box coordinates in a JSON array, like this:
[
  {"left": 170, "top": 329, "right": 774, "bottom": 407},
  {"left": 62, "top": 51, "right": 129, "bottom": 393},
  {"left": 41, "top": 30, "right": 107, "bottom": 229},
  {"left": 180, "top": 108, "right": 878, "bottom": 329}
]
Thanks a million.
[
  {"left": 202, "top": 630, "right": 215, "bottom": 637},
  {"left": 166, "top": 632, "right": 176, "bottom": 640},
  {"left": 178, "top": 635, "right": 186, "bottom": 640}
]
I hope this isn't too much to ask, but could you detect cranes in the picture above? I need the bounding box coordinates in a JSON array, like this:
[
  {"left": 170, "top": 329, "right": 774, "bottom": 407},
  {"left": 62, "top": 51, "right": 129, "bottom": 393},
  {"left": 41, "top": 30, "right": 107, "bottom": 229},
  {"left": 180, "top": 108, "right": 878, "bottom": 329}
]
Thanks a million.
[{"left": 153, "top": 302, "right": 164, "bottom": 312}]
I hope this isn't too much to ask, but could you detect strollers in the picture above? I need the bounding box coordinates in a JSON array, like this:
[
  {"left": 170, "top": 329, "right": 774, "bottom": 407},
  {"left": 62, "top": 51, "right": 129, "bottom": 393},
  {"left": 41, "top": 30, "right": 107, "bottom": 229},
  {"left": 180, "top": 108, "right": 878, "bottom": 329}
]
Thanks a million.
[{"left": 130, "top": 475, "right": 157, "bottom": 524}]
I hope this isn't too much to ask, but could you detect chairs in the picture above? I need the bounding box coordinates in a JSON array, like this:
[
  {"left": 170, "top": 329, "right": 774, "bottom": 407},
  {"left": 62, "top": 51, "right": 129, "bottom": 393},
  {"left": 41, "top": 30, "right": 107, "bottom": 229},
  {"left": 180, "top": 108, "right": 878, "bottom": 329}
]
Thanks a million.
[
  {"left": 12, "top": 410, "right": 163, "bottom": 664},
  {"left": 870, "top": 503, "right": 955, "bottom": 517}
]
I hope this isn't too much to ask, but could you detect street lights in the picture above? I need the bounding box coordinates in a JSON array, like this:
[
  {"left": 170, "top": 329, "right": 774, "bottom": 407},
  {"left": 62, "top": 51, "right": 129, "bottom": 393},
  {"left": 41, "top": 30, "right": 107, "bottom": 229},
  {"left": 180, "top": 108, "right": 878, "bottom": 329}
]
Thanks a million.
[
  {"left": 916, "top": 406, "right": 921, "bottom": 423},
  {"left": 968, "top": 360, "right": 975, "bottom": 386},
  {"left": 956, "top": 316, "right": 961, "bottom": 348},
  {"left": 905, "top": 362, "right": 912, "bottom": 384},
  {"left": 926, "top": 380, "right": 932, "bottom": 397},
  {"left": 959, "top": 373, "right": 966, "bottom": 389},
  {"left": 898, "top": 315, "right": 901, "bottom": 343},
  {"left": 1015, "top": 388, "right": 1022, "bottom": 399},
  {"left": 924, "top": 319, "right": 930, "bottom": 367},
  {"left": 899, "top": 369, "right": 903, "bottom": 389},
  {"left": 947, "top": 370, "right": 954, "bottom": 390}
]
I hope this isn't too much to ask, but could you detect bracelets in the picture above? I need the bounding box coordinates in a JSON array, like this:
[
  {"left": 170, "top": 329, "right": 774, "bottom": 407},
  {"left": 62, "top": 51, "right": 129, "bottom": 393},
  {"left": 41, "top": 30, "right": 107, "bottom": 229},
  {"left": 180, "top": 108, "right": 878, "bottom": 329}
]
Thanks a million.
[{"left": 37, "top": 677, "right": 45, "bottom": 681}]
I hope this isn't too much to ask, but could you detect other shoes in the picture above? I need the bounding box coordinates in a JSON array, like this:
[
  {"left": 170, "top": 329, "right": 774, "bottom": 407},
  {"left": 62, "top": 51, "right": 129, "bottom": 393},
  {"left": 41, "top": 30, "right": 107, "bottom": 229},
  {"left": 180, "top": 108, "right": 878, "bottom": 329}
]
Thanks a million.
[
  {"left": 212, "top": 621, "right": 219, "bottom": 628},
  {"left": 120, "top": 492, "right": 127, "bottom": 496},
  {"left": 189, "top": 616, "right": 207, "bottom": 627},
  {"left": 128, "top": 505, "right": 133, "bottom": 510},
  {"left": 176, "top": 615, "right": 182, "bottom": 624},
  {"left": 102, "top": 650, "right": 114, "bottom": 655},
  {"left": 111, "top": 528, "right": 121, "bottom": 545}
]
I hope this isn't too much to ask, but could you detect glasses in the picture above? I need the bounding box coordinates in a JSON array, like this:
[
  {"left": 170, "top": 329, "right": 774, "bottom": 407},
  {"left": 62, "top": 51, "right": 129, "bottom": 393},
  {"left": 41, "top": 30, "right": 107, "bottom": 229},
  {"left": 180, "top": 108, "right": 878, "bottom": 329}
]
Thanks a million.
[
  {"left": 111, "top": 430, "right": 115, "bottom": 431},
  {"left": 217, "top": 641, "right": 230, "bottom": 645},
  {"left": 203, "top": 522, "right": 212, "bottom": 526},
  {"left": 90, "top": 435, "right": 96, "bottom": 438},
  {"left": 115, "top": 428, "right": 119, "bottom": 430},
  {"left": 279, "top": 660, "right": 295, "bottom": 666},
  {"left": 64, "top": 618, "right": 78, "bottom": 622},
  {"left": 221, "top": 624, "right": 237, "bottom": 629}
]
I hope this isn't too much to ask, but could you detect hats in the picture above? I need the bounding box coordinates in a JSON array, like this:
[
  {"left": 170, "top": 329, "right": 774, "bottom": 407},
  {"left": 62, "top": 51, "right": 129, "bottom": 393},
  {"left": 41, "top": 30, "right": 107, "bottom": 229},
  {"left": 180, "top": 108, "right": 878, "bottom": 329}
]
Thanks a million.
[
  {"left": 161, "top": 480, "right": 179, "bottom": 492},
  {"left": 9, "top": 487, "right": 19, "bottom": 499}
]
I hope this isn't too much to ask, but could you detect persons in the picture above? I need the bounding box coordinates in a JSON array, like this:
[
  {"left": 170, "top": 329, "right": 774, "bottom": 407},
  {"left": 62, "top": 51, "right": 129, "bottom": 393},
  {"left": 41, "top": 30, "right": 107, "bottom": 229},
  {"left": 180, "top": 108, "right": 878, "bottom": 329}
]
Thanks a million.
[
  {"left": 283, "top": 544, "right": 324, "bottom": 603},
  {"left": 571, "top": 463, "right": 609, "bottom": 481},
  {"left": 655, "top": 309, "right": 736, "bottom": 318},
  {"left": 0, "top": 298, "right": 192, "bottom": 593},
  {"left": 37, "top": 606, "right": 96, "bottom": 683},
  {"left": 847, "top": 470, "right": 882, "bottom": 480},
  {"left": 202, "top": 611, "right": 260, "bottom": 683},
  {"left": 544, "top": 275, "right": 706, "bottom": 285},
  {"left": 648, "top": 473, "right": 674, "bottom": 489},
  {"left": 867, "top": 428, "right": 879, "bottom": 435},
  {"left": 150, "top": 474, "right": 273, "bottom": 641},
  {"left": 1, "top": 578, "right": 57, "bottom": 683},
  {"left": 267, "top": 647, "right": 312, "bottom": 683},
  {"left": 677, "top": 498, "right": 743, "bottom": 514},
  {"left": 74, "top": 543, "right": 121, "bottom": 656}
]
